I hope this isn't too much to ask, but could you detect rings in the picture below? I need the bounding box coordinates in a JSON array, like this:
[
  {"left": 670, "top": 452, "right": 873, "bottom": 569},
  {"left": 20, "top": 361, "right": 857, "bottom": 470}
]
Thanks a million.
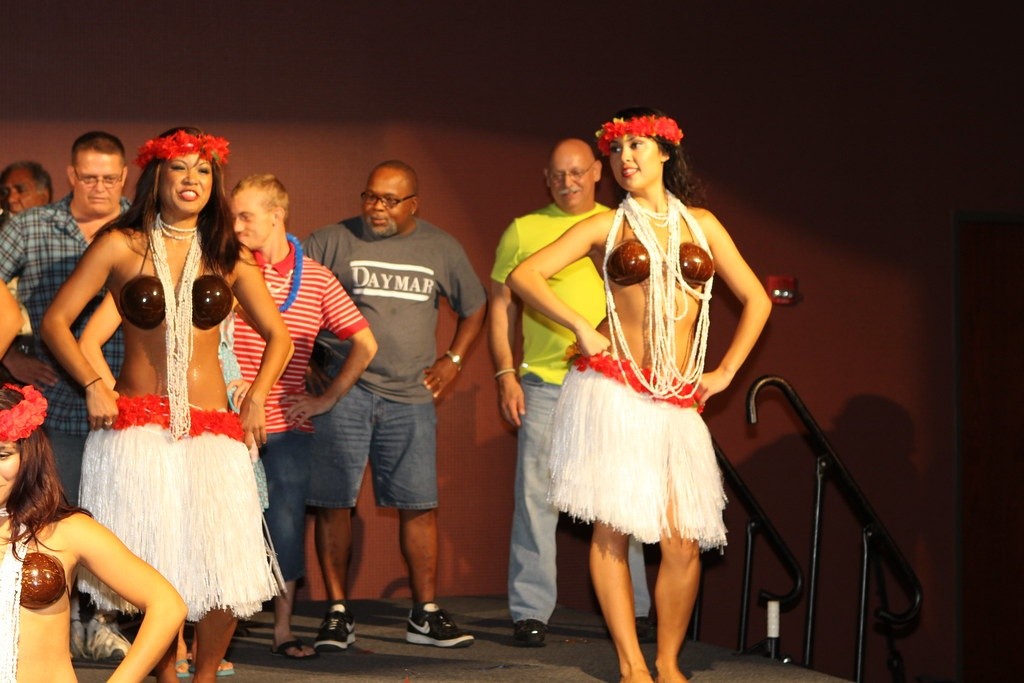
[
  {"left": 435, "top": 377, "right": 440, "bottom": 382},
  {"left": 241, "top": 394, "right": 246, "bottom": 398},
  {"left": 104, "top": 421, "right": 112, "bottom": 427}
]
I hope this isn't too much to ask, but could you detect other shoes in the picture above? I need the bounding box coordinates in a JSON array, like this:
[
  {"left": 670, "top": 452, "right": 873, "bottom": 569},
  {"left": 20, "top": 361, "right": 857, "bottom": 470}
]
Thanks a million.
[
  {"left": 513, "top": 619, "right": 546, "bottom": 647},
  {"left": 634, "top": 617, "right": 656, "bottom": 643}
]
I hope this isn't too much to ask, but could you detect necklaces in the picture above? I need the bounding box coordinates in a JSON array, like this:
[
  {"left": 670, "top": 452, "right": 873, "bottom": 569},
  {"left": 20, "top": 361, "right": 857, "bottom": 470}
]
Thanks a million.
[
  {"left": 267, "top": 270, "right": 293, "bottom": 294},
  {"left": 276, "top": 234, "right": 303, "bottom": 312},
  {"left": 0, "top": 507, "right": 31, "bottom": 683},
  {"left": 603, "top": 190, "right": 715, "bottom": 397},
  {"left": 149, "top": 213, "right": 202, "bottom": 441}
]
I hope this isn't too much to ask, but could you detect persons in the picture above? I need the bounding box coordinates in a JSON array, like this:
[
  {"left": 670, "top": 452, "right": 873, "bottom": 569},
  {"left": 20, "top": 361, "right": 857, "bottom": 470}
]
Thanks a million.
[
  {"left": 0, "top": 385, "right": 186, "bottom": 683},
  {"left": 487, "top": 140, "right": 658, "bottom": 645},
  {"left": 233, "top": 173, "right": 378, "bottom": 662},
  {"left": 78, "top": 290, "right": 295, "bottom": 678},
  {"left": 299, "top": 161, "right": 489, "bottom": 650},
  {"left": 505, "top": 103, "right": 773, "bottom": 683},
  {"left": 42, "top": 126, "right": 295, "bottom": 683},
  {"left": 0, "top": 131, "right": 133, "bottom": 666}
]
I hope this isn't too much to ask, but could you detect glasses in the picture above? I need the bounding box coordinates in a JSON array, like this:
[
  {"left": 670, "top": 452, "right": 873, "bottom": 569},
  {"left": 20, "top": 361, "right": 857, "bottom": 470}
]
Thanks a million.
[
  {"left": 548, "top": 161, "right": 595, "bottom": 184},
  {"left": 73, "top": 167, "right": 124, "bottom": 188},
  {"left": 360, "top": 190, "right": 417, "bottom": 209}
]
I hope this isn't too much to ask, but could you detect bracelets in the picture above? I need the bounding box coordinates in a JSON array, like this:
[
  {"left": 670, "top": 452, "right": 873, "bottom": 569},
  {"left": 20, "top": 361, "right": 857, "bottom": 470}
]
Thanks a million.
[
  {"left": 495, "top": 369, "right": 515, "bottom": 380},
  {"left": 445, "top": 350, "right": 461, "bottom": 366},
  {"left": 83, "top": 377, "right": 102, "bottom": 389}
]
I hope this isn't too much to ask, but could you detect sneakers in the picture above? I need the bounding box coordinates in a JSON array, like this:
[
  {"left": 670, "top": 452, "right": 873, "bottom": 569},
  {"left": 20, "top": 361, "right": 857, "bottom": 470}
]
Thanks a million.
[
  {"left": 406, "top": 601, "right": 474, "bottom": 646},
  {"left": 70, "top": 596, "right": 130, "bottom": 664},
  {"left": 313, "top": 601, "right": 356, "bottom": 650}
]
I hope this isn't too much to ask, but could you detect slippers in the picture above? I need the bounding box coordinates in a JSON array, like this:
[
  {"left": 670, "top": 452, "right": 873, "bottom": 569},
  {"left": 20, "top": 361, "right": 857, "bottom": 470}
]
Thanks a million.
[
  {"left": 269, "top": 635, "right": 318, "bottom": 659},
  {"left": 188, "top": 659, "right": 236, "bottom": 676},
  {"left": 176, "top": 658, "right": 190, "bottom": 678}
]
